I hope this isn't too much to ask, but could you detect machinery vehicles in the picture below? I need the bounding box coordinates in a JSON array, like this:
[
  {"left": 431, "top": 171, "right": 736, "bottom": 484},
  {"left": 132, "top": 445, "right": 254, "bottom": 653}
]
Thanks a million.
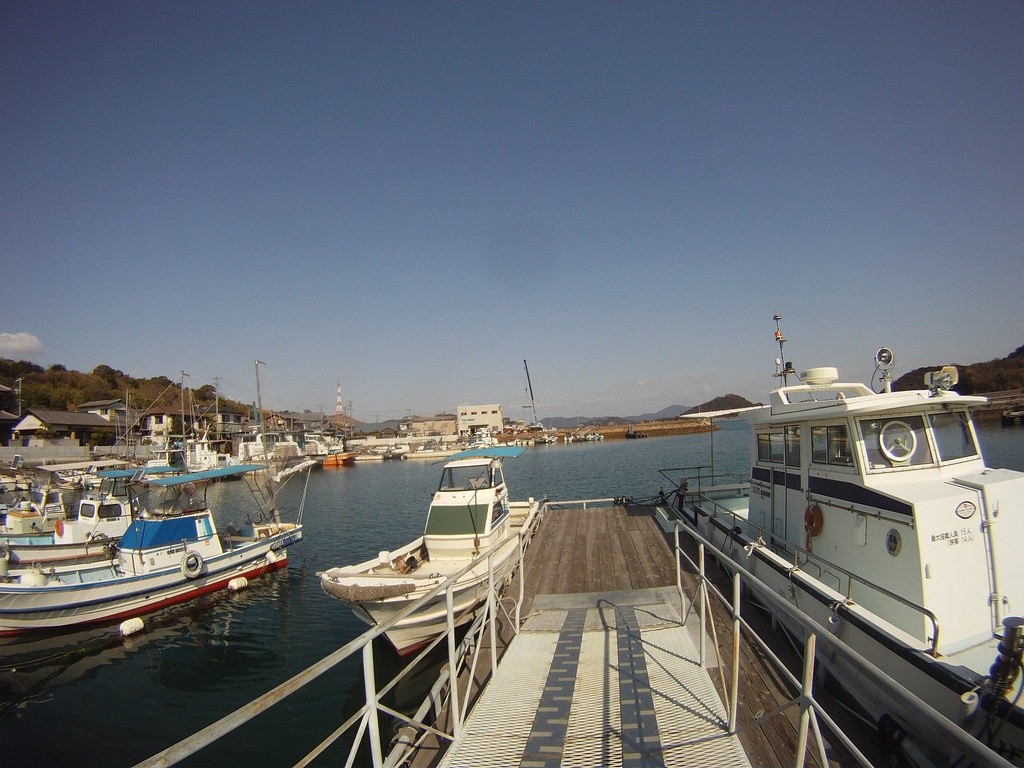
[{"left": 522, "top": 359, "right": 543, "bottom": 432}]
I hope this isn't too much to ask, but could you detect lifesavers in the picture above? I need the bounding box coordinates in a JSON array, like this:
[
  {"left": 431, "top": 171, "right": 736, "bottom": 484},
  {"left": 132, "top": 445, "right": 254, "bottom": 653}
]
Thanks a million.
[
  {"left": 55, "top": 520, "right": 63, "bottom": 536},
  {"left": 802, "top": 504, "right": 824, "bottom": 537},
  {"left": 178, "top": 550, "right": 206, "bottom": 580}
]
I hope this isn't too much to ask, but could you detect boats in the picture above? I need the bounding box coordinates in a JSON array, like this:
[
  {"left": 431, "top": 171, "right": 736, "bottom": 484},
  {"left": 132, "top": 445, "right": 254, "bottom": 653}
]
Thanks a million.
[
  {"left": 0, "top": 439, "right": 311, "bottom": 637},
  {"left": 657, "top": 311, "right": 1023, "bottom": 767},
  {"left": 315, "top": 442, "right": 548, "bottom": 656}
]
